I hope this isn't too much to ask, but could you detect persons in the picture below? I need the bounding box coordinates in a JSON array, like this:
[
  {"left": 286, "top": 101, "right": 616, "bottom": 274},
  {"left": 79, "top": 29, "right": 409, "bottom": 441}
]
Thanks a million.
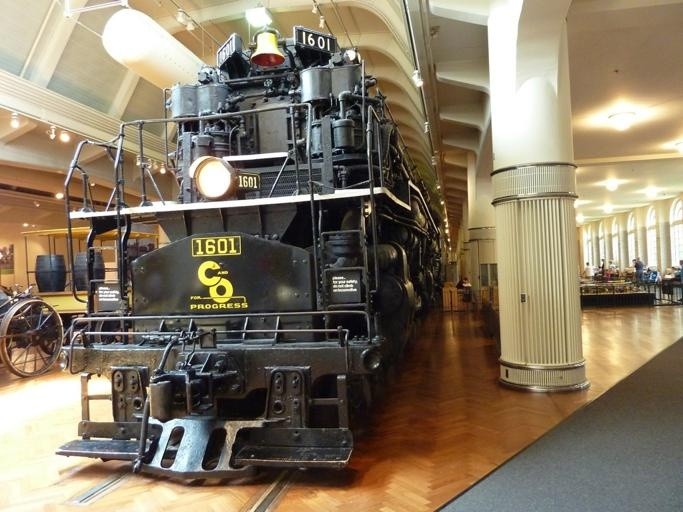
[
  {"left": 608, "top": 260, "right": 617, "bottom": 269},
  {"left": 677, "top": 259, "right": 683, "bottom": 302},
  {"left": 635, "top": 256, "right": 648, "bottom": 290},
  {"left": 597, "top": 258, "right": 604, "bottom": 275},
  {"left": 582, "top": 262, "right": 595, "bottom": 280},
  {"left": 631, "top": 258, "right": 637, "bottom": 280},
  {"left": 456, "top": 278, "right": 476, "bottom": 303},
  {"left": 670, "top": 266, "right": 678, "bottom": 278}
]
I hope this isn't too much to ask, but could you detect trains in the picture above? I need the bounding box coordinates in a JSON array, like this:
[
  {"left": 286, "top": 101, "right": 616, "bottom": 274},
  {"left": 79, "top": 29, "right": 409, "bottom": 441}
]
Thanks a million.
[{"left": 53, "top": 24, "right": 450, "bottom": 481}]
